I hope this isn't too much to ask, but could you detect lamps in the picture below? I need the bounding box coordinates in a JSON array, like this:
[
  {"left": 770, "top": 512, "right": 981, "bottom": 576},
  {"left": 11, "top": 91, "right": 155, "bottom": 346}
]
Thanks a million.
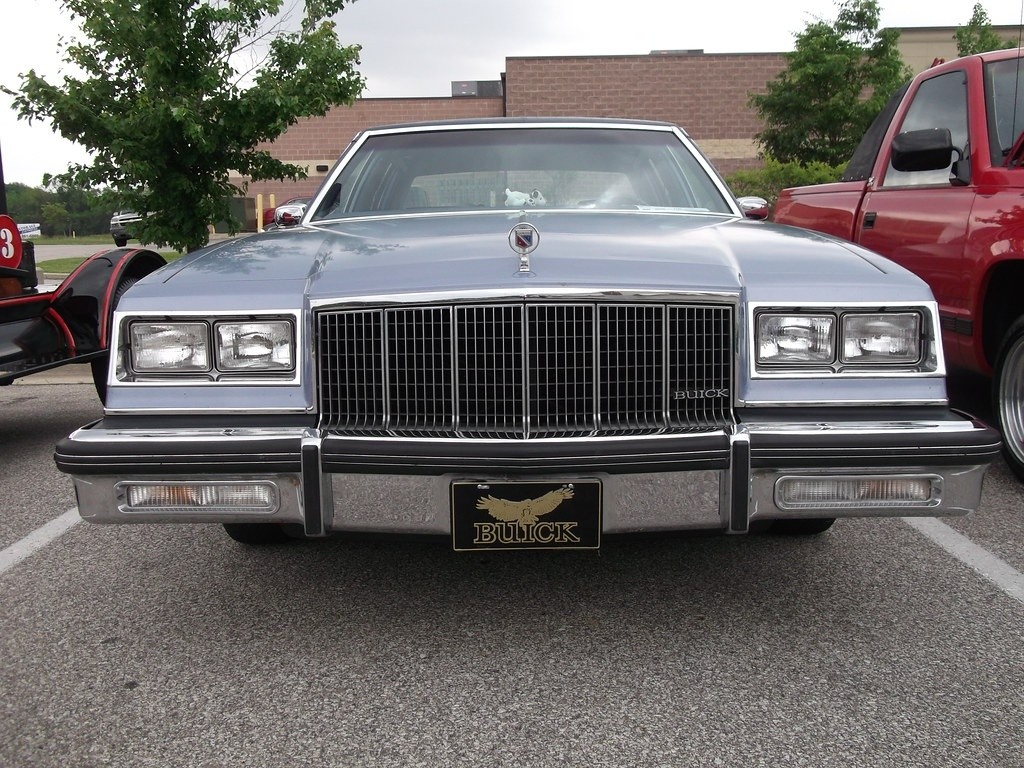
[{"left": 317, "top": 165, "right": 328, "bottom": 171}]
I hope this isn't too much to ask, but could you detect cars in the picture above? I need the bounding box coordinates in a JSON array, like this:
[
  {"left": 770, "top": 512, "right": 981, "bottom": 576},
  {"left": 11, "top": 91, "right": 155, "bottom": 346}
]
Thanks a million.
[
  {"left": 255, "top": 194, "right": 313, "bottom": 227},
  {"left": 50, "top": 111, "right": 1005, "bottom": 555},
  {"left": 0, "top": 147, "right": 168, "bottom": 407}
]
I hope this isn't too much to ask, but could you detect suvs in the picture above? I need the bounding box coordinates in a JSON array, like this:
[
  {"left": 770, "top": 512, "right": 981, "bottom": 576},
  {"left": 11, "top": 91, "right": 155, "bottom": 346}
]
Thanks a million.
[{"left": 109, "top": 208, "right": 158, "bottom": 247}]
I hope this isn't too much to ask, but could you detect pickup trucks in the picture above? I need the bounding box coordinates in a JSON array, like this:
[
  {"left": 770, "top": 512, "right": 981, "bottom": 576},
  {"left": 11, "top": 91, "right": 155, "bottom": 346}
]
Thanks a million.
[{"left": 772, "top": 45, "right": 1024, "bottom": 487}]
